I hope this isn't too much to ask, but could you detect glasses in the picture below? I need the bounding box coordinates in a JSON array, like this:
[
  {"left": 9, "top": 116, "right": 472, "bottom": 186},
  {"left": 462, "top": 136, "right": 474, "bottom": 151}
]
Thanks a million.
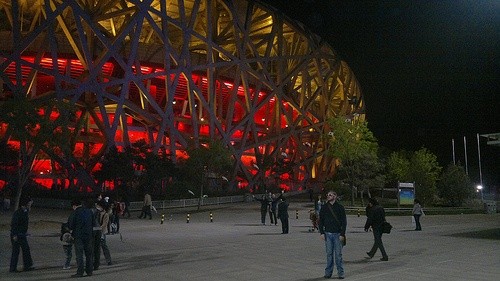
[{"left": 326, "top": 194, "right": 337, "bottom": 198}]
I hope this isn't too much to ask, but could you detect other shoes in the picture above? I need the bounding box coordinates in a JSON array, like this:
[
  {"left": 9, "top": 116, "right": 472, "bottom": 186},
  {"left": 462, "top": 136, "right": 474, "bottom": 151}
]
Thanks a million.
[
  {"left": 415, "top": 228, "right": 421, "bottom": 230},
  {"left": 275, "top": 224, "right": 278, "bottom": 226},
  {"left": 324, "top": 273, "right": 332, "bottom": 278},
  {"left": 63, "top": 265, "right": 72, "bottom": 269},
  {"left": 366, "top": 252, "right": 372, "bottom": 259},
  {"left": 380, "top": 256, "right": 388, "bottom": 261},
  {"left": 270, "top": 223, "right": 274, "bottom": 225},
  {"left": 339, "top": 276, "right": 344, "bottom": 279}
]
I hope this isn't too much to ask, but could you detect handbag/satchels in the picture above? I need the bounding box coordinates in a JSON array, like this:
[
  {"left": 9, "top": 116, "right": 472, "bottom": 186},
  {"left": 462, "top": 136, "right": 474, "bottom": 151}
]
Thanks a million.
[
  {"left": 419, "top": 205, "right": 425, "bottom": 216},
  {"left": 152, "top": 206, "right": 158, "bottom": 216},
  {"left": 343, "top": 235, "right": 346, "bottom": 246},
  {"left": 382, "top": 219, "right": 392, "bottom": 234}
]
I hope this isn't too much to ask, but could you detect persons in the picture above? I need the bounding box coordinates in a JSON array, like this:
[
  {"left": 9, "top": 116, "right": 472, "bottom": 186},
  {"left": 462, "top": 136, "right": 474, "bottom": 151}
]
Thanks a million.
[
  {"left": 269, "top": 194, "right": 278, "bottom": 225},
  {"left": 96, "top": 202, "right": 112, "bottom": 265},
  {"left": 267, "top": 192, "right": 273, "bottom": 212},
  {"left": 318, "top": 192, "right": 348, "bottom": 279},
  {"left": 278, "top": 197, "right": 291, "bottom": 234},
  {"left": 104, "top": 197, "right": 115, "bottom": 235},
  {"left": 261, "top": 195, "right": 267, "bottom": 224},
  {"left": 95, "top": 193, "right": 103, "bottom": 202},
  {"left": 60, "top": 223, "right": 73, "bottom": 270},
  {"left": 85, "top": 202, "right": 101, "bottom": 270},
  {"left": 122, "top": 191, "right": 131, "bottom": 217},
  {"left": 366, "top": 197, "right": 379, "bottom": 217},
  {"left": 363, "top": 197, "right": 391, "bottom": 261},
  {"left": 68, "top": 201, "right": 92, "bottom": 276},
  {"left": 113, "top": 196, "right": 124, "bottom": 234},
  {"left": 314, "top": 194, "right": 326, "bottom": 232},
  {"left": 412, "top": 198, "right": 425, "bottom": 231},
  {"left": 144, "top": 191, "right": 153, "bottom": 220},
  {"left": 10, "top": 198, "right": 34, "bottom": 272}
]
